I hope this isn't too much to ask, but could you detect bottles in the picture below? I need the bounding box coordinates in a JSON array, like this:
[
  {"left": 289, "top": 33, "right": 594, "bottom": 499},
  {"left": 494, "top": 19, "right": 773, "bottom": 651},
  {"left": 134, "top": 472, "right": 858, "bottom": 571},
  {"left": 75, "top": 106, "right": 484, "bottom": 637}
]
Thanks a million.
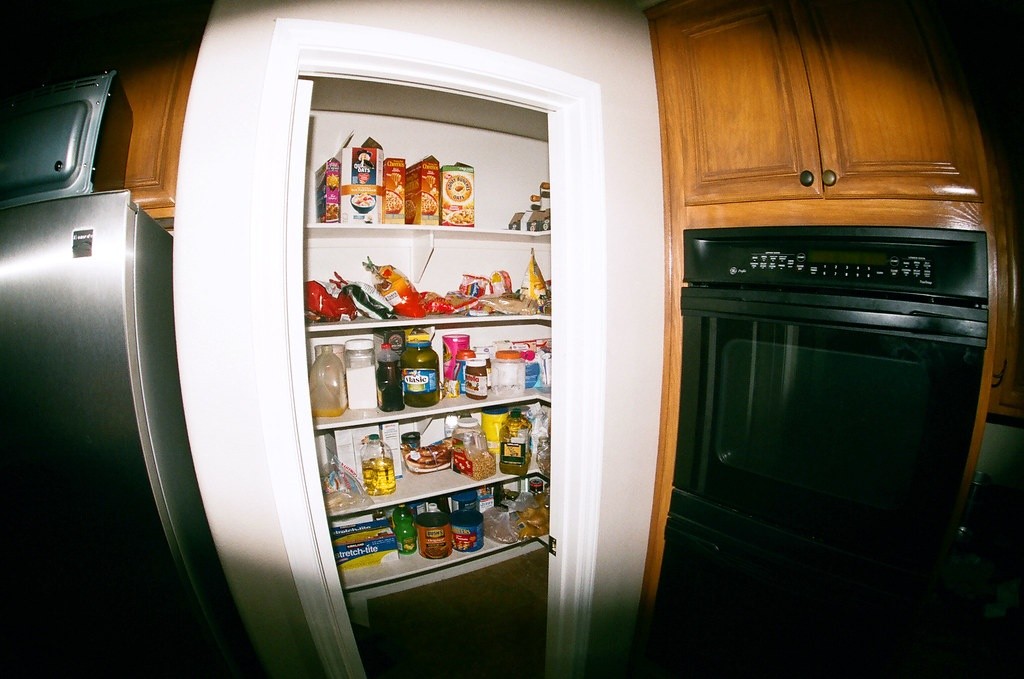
[
  {"left": 452, "top": 418, "right": 496, "bottom": 481},
  {"left": 500, "top": 408, "right": 532, "bottom": 475},
  {"left": 491, "top": 350, "right": 525, "bottom": 397},
  {"left": 392, "top": 504, "right": 416, "bottom": 555},
  {"left": 360, "top": 434, "right": 396, "bottom": 495},
  {"left": 536, "top": 437, "right": 550, "bottom": 476},
  {"left": 375, "top": 344, "right": 404, "bottom": 412},
  {"left": 310, "top": 345, "right": 348, "bottom": 418}
]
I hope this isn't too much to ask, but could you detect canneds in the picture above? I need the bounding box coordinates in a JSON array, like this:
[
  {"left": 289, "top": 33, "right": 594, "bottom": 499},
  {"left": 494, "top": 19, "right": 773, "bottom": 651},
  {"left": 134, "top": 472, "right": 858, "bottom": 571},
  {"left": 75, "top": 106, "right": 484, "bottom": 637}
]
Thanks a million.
[
  {"left": 465, "top": 358, "right": 488, "bottom": 399},
  {"left": 475, "top": 355, "right": 492, "bottom": 389},
  {"left": 415, "top": 512, "right": 452, "bottom": 559},
  {"left": 449, "top": 510, "right": 483, "bottom": 552}
]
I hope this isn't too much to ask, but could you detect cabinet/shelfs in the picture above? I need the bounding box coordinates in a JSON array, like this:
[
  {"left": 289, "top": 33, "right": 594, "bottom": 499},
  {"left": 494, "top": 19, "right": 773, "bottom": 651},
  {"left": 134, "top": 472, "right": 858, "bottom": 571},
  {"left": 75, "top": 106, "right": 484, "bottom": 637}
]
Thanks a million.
[
  {"left": 671, "top": 0, "right": 983, "bottom": 206},
  {"left": 303, "top": 223, "right": 553, "bottom": 629}
]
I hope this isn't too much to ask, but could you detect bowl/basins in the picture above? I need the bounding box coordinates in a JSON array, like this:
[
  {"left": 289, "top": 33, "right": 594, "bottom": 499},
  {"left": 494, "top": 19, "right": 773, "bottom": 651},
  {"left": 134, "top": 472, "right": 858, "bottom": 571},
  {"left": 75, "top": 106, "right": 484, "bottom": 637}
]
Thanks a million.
[
  {"left": 385, "top": 190, "right": 403, "bottom": 214},
  {"left": 422, "top": 191, "right": 437, "bottom": 215},
  {"left": 351, "top": 195, "right": 376, "bottom": 213}
]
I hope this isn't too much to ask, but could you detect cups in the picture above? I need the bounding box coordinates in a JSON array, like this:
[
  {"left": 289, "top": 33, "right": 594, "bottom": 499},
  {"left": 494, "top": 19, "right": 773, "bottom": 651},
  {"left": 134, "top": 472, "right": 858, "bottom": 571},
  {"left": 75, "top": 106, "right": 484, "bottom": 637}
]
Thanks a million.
[{"left": 345, "top": 339, "right": 374, "bottom": 409}]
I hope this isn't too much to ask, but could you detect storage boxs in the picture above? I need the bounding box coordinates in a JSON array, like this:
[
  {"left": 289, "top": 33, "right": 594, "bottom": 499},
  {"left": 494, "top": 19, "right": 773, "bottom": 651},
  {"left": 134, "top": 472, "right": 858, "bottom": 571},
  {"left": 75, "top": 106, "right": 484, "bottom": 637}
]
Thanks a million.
[
  {"left": 405, "top": 154, "right": 439, "bottom": 225},
  {"left": 315, "top": 422, "right": 403, "bottom": 480},
  {"left": 314, "top": 157, "right": 340, "bottom": 223},
  {"left": 328, "top": 513, "right": 400, "bottom": 572},
  {"left": 383, "top": 158, "right": 405, "bottom": 223},
  {"left": 509, "top": 209, "right": 551, "bottom": 232},
  {"left": 334, "top": 130, "right": 382, "bottom": 224},
  {"left": 439, "top": 161, "right": 475, "bottom": 227}
]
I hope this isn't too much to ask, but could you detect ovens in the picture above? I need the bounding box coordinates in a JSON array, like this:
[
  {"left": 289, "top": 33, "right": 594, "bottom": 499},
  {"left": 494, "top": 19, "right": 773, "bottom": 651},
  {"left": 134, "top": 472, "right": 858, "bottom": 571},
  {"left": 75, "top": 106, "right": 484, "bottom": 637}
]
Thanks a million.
[{"left": 643, "top": 225, "right": 988, "bottom": 679}]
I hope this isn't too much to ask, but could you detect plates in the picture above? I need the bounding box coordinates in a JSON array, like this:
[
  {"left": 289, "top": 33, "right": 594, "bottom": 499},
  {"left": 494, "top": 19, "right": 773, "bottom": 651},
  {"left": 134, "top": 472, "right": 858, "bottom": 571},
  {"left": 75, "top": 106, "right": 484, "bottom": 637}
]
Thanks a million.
[{"left": 447, "top": 210, "right": 474, "bottom": 224}]
